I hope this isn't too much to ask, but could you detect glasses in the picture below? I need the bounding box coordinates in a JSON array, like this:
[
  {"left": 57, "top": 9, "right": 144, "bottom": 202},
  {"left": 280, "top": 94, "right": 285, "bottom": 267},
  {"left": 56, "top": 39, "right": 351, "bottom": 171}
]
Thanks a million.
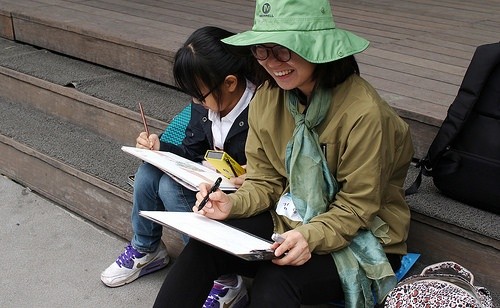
[
  {"left": 193, "top": 82, "right": 222, "bottom": 105},
  {"left": 251, "top": 46, "right": 291, "bottom": 62}
]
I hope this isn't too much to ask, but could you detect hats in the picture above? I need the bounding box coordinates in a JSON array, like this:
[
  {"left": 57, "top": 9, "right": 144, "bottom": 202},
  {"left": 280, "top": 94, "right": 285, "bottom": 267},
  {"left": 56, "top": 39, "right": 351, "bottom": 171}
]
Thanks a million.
[{"left": 220, "top": 0, "right": 371, "bottom": 64}]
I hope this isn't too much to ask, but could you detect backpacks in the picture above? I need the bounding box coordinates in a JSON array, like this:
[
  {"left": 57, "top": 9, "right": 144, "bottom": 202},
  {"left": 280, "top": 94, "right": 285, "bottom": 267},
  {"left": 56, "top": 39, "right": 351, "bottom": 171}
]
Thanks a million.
[{"left": 405, "top": 42, "right": 500, "bottom": 216}]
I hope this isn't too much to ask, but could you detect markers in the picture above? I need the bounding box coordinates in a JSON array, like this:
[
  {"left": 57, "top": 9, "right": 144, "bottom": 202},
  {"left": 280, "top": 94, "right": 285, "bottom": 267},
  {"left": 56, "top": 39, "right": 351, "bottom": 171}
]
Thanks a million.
[{"left": 271, "top": 233, "right": 285, "bottom": 245}]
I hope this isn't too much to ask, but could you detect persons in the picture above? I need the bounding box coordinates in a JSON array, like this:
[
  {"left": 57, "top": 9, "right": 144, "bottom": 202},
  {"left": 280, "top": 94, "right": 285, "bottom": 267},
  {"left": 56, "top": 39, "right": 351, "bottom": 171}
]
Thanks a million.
[
  {"left": 101, "top": 26, "right": 279, "bottom": 308},
  {"left": 192, "top": 0, "right": 413, "bottom": 308}
]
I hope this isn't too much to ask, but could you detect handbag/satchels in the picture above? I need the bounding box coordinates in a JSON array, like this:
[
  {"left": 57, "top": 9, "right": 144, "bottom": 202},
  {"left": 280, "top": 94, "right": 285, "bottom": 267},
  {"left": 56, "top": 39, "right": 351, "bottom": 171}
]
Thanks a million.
[{"left": 383, "top": 261, "right": 494, "bottom": 308}]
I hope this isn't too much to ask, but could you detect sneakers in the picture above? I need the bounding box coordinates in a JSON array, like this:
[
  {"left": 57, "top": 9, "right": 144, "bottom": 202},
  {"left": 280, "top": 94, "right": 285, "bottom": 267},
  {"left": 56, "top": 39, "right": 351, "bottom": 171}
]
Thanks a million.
[
  {"left": 100, "top": 240, "right": 169, "bottom": 287},
  {"left": 202, "top": 274, "right": 248, "bottom": 308}
]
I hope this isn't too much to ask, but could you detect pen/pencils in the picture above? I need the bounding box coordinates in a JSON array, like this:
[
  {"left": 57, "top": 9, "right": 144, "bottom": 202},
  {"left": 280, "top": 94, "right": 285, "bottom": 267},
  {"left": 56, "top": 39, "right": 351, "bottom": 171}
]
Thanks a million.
[
  {"left": 196, "top": 176, "right": 222, "bottom": 211},
  {"left": 138, "top": 102, "right": 154, "bottom": 151}
]
